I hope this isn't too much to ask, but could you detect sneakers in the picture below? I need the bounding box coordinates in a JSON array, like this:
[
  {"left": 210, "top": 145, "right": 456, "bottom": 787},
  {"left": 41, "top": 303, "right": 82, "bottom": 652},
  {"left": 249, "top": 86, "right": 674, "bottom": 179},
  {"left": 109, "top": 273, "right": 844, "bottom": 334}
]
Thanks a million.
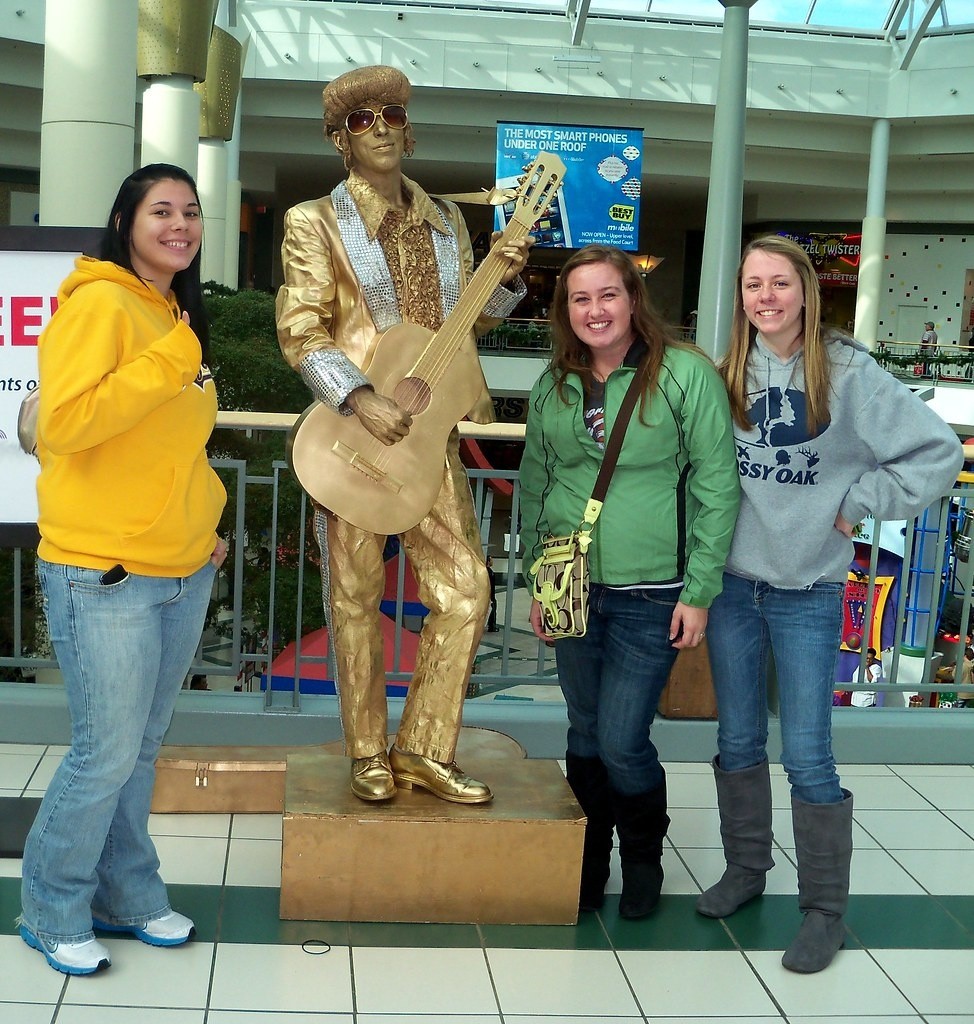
[
  {"left": 91, "top": 910, "right": 195, "bottom": 946},
  {"left": 20, "top": 924, "right": 111, "bottom": 974}
]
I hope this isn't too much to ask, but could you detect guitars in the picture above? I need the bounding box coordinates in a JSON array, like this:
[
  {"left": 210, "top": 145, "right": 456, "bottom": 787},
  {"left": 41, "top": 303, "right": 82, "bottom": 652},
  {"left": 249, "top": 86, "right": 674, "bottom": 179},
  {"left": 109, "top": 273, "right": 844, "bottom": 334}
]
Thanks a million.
[{"left": 281, "top": 151, "right": 570, "bottom": 539}]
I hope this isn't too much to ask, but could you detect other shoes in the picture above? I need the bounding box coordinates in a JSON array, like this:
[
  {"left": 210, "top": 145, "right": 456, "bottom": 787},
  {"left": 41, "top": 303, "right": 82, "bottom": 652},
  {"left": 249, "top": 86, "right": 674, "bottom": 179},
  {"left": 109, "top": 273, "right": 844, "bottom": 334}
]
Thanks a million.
[{"left": 487, "top": 627, "right": 499, "bottom": 632}]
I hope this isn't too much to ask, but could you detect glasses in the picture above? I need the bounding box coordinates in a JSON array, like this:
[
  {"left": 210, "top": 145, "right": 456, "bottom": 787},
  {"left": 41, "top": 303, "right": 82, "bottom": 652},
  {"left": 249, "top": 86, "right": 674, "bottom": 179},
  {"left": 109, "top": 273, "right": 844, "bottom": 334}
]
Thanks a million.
[{"left": 339, "top": 105, "right": 410, "bottom": 135}]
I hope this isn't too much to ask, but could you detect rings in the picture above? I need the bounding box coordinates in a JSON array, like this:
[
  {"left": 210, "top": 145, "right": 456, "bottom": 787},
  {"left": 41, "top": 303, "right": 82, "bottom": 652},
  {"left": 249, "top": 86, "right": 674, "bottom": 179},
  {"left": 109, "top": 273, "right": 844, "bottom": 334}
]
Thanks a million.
[{"left": 700, "top": 633, "right": 705, "bottom": 637}]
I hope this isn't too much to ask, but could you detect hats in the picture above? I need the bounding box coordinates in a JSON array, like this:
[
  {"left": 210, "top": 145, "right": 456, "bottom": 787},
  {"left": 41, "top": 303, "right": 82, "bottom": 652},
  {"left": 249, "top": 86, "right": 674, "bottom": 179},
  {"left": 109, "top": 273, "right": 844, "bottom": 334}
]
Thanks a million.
[{"left": 924, "top": 322, "right": 934, "bottom": 329}]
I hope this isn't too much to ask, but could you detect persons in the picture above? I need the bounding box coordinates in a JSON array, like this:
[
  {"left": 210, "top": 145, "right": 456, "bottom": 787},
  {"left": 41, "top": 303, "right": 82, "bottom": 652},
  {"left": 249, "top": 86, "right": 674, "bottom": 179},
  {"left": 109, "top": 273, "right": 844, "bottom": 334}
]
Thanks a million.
[
  {"left": 517, "top": 244, "right": 743, "bottom": 918},
  {"left": 276, "top": 66, "right": 536, "bottom": 804},
  {"left": 919, "top": 322, "right": 937, "bottom": 379},
  {"left": 851, "top": 648, "right": 882, "bottom": 707},
  {"left": 19, "top": 163, "right": 228, "bottom": 975},
  {"left": 190, "top": 673, "right": 208, "bottom": 690},
  {"left": 696, "top": 236, "right": 964, "bottom": 972},
  {"left": 486, "top": 555, "right": 500, "bottom": 632},
  {"left": 952, "top": 648, "right": 974, "bottom": 708}
]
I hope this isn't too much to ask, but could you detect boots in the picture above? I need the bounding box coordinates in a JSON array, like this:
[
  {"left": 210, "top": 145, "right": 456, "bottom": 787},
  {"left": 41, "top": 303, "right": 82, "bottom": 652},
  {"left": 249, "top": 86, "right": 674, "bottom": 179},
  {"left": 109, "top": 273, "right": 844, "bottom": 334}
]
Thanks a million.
[
  {"left": 695, "top": 754, "right": 775, "bottom": 917},
  {"left": 612, "top": 768, "right": 670, "bottom": 920},
  {"left": 782, "top": 787, "right": 853, "bottom": 973},
  {"left": 564, "top": 751, "right": 614, "bottom": 912}
]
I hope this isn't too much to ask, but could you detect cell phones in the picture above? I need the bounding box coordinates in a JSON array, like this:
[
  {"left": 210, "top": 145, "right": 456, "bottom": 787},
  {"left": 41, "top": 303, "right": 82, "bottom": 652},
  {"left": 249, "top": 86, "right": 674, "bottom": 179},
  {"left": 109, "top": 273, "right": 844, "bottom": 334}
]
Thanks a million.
[{"left": 99, "top": 563, "right": 128, "bottom": 585}]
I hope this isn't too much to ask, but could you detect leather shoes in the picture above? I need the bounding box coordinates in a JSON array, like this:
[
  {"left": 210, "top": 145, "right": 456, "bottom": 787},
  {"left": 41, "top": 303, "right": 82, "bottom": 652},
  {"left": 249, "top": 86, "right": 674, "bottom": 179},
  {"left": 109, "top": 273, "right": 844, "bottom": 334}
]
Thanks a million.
[
  {"left": 388, "top": 743, "right": 494, "bottom": 803},
  {"left": 351, "top": 750, "right": 398, "bottom": 800}
]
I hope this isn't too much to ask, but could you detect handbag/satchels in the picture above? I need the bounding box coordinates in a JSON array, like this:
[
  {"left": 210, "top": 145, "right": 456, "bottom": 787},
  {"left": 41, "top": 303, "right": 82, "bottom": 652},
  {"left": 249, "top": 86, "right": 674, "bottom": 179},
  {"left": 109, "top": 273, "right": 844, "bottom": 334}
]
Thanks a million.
[
  {"left": 530, "top": 530, "right": 593, "bottom": 641},
  {"left": 914, "top": 364, "right": 923, "bottom": 376}
]
